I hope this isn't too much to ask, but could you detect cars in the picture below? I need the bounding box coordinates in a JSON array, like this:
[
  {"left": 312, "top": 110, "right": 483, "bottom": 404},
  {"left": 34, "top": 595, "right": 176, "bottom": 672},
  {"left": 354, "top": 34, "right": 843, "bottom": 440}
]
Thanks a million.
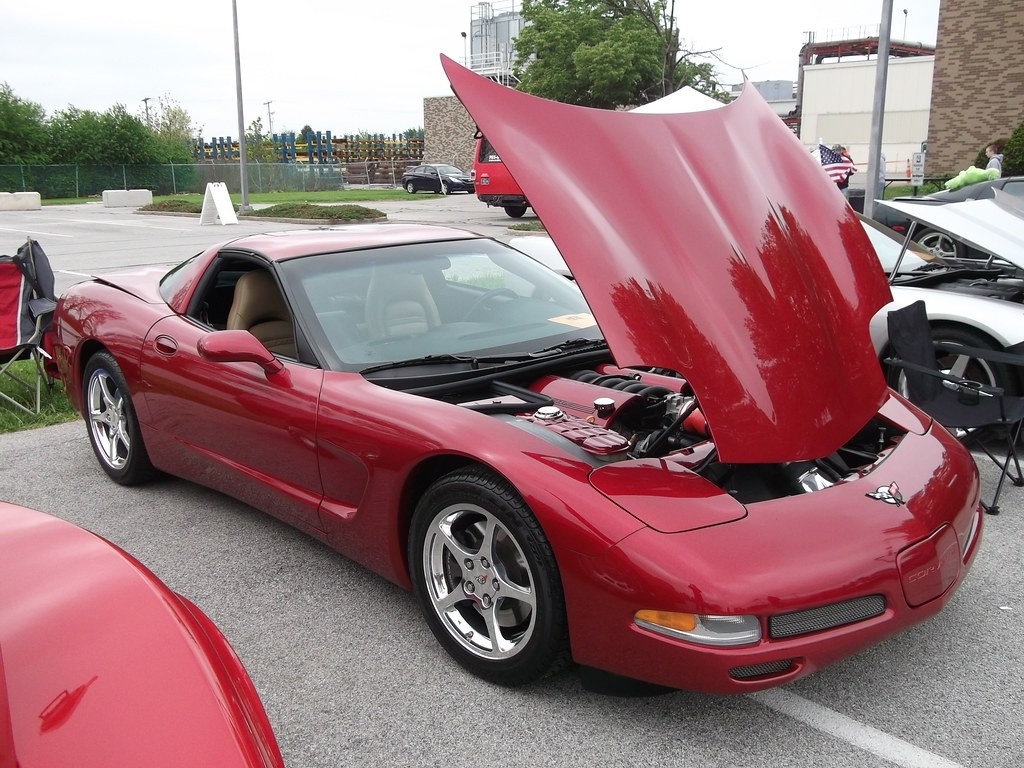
[
  {"left": 399, "top": 162, "right": 475, "bottom": 196},
  {"left": 873, "top": 174, "right": 1024, "bottom": 275}
]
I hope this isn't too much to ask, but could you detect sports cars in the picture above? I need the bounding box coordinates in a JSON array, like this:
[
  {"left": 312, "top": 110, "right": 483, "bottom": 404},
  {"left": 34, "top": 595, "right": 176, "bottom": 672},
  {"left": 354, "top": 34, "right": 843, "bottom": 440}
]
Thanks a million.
[
  {"left": 51, "top": 50, "right": 984, "bottom": 698},
  {"left": 0, "top": 500, "right": 285, "bottom": 768},
  {"left": 446, "top": 187, "right": 1024, "bottom": 452}
]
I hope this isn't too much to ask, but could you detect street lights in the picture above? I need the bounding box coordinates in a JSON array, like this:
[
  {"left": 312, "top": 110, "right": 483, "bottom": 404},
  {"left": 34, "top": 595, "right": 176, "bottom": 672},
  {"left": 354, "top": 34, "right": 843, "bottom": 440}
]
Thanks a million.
[
  {"left": 460, "top": 31, "right": 468, "bottom": 68},
  {"left": 901, "top": 9, "right": 910, "bottom": 42},
  {"left": 140, "top": 97, "right": 151, "bottom": 126}
]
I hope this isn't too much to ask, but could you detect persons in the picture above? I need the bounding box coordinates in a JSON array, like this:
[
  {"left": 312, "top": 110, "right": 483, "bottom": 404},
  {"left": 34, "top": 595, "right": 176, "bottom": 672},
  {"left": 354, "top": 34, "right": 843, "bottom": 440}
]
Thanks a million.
[
  {"left": 830, "top": 144, "right": 856, "bottom": 202},
  {"left": 985, "top": 145, "right": 1004, "bottom": 179},
  {"left": 877, "top": 155, "right": 887, "bottom": 199}
]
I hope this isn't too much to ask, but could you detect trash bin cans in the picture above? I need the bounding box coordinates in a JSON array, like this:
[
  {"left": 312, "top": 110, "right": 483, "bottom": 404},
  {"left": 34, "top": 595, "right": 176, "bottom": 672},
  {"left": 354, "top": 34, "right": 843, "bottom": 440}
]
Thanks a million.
[{"left": 845, "top": 188, "right": 865, "bottom": 214}]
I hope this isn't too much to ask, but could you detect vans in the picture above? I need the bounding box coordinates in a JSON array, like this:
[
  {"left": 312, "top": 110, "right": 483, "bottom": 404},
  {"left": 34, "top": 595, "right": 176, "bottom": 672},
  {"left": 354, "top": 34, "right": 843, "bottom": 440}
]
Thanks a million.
[{"left": 468, "top": 130, "right": 538, "bottom": 218}]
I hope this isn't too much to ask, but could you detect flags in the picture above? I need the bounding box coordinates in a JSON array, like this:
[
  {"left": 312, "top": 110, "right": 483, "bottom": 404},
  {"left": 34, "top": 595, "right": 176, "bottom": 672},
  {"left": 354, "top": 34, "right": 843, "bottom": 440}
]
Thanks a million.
[{"left": 811, "top": 145, "right": 858, "bottom": 184}]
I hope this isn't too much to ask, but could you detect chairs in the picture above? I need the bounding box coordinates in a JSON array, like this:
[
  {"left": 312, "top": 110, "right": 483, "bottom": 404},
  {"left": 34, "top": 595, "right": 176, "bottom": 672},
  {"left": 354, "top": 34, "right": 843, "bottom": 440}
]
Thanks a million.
[
  {"left": 223, "top": 267, "right": 297, "bottom": 364},
  {"left": 0, "top": 235, "right": 58, "bottom": 415},
  {"left": 365, "top": 262, "right": 444, "bottom": 341},
  {"left": 884, "top": 299, "right": 1024, "bottom": 517}
]
